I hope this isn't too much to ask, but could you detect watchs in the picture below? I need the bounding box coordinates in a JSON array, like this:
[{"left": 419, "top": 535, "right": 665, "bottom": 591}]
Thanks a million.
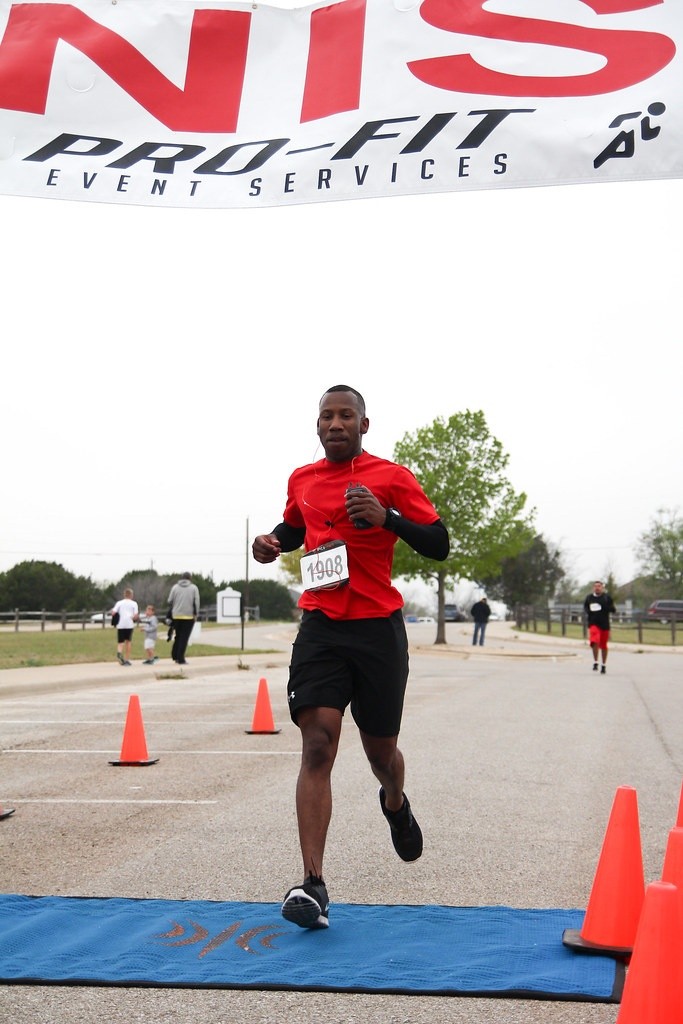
[{"left": 386, "top": 507, "right": 401, "bottom": 529}]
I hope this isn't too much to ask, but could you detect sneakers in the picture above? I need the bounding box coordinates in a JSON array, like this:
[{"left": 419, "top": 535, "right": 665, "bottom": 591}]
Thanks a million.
[
  {"left": 281, "top": 855, "right": 330, "bottom": 928},
  {"left": 379, "top": 785, "right": 424, "bottom": 861}
]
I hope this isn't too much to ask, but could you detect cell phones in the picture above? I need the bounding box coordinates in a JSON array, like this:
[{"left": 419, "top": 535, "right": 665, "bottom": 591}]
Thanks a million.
[{"left": 345, "top": 488, "right": 374, "bottom": 528}]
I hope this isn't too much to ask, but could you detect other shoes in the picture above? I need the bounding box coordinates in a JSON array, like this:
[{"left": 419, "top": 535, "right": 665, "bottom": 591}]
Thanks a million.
[
  {"left": 601, "top": 665, "right": 606, "bottom": 675},
  {"left": 593, "top": 663, "right": 598, "bottom": 671},
  {"left": 145, "top": 657, "right": 154, "bottom": 664},
  {"left": 117, "top": 654, "right": 130, "bottom": 665}
]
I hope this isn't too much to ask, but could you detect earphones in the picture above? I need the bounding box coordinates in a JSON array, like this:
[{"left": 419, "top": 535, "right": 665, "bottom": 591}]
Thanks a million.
[{"left": 325, "top": 520, "right": 334, "bottom": 528}]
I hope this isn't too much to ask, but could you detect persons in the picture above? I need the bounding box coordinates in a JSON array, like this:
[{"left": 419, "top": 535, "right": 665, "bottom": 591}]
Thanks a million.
[
  {"left": 251, "top": 385, "right": 451, "bottom": 929},
  {"left": 138, "top": 605, "right": 159, "bottom": 665},
  {"left": 166, "top": 607, "right": 177, "bottom": 642},
  {"left": 470, "top": 598, "right": 491, "bottom": 646},
  {"left": 111, "top": 588, "right": 138, "bottom": 665},
  {"left": 167, "top": 571, "right": 201, "bottom": 664},
  {"left": 583, "top": 581, "right": 617, "bottom": 674}
]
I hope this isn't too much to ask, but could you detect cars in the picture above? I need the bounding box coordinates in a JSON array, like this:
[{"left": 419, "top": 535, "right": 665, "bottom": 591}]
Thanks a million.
[
  {"left": 646, "top": 598, "right": 683, "bottom": 624},
  {"left": 405, "top": 603, "right": 501, "bottom": 623},
  {"left": 90, "top": 606, "right": 147, "bottom": 624}
]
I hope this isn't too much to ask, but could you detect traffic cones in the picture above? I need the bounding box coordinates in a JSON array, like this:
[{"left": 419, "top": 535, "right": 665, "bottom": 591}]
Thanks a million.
[
  {"left": 614, "top": 779, "right": 683, "bottom": 1024},
  {"left": 108, "top": 694, "right": 160, "bottom": 765},
  {"left": 245, "top": 676, "right": 282, "bottom": 734},
  {"left": 561, "top": 782, "right": 647, "bottom": 959}
]
